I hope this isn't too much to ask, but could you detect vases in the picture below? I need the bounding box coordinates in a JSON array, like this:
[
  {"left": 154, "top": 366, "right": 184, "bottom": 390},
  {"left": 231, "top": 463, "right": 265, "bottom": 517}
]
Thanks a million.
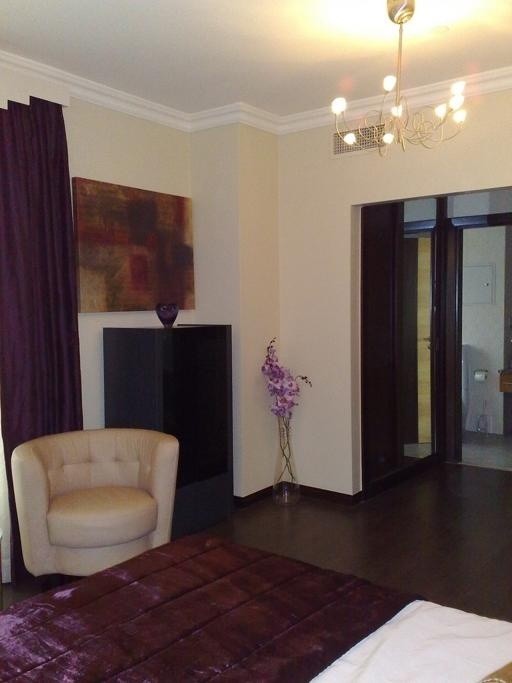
[{"left": 273, "top": 414, "right": 300, "bottom": 507}]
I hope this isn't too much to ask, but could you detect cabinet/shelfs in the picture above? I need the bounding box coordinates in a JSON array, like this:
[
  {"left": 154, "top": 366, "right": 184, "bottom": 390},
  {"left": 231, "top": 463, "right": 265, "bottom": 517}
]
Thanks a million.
[{"left": 104, "top": 323, "right": 233, "bottom": 488}]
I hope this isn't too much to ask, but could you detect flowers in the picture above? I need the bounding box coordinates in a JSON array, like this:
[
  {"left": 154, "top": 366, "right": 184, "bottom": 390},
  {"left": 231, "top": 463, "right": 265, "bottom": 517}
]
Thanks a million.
[{"left": 262, "top": 336, "right": 312, "bottom": 418}]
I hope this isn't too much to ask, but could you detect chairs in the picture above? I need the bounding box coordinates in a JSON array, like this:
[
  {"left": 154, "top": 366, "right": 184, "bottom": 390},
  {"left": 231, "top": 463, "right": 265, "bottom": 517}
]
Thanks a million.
[{"left": 10, "top": 427, "right": 180, "bottom": 578}]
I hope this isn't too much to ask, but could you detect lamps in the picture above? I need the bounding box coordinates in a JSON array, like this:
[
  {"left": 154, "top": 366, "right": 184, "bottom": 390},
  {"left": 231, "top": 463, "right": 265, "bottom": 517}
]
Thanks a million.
[{"left": 332, "top": 0, "right": 471, "bottom": 158}]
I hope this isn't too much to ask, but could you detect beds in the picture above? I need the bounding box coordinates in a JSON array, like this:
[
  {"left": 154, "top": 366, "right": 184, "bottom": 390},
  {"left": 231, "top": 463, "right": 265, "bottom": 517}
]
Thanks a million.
[{"left": 0, "top": 533, "right": 512, "bottom": 683}]
